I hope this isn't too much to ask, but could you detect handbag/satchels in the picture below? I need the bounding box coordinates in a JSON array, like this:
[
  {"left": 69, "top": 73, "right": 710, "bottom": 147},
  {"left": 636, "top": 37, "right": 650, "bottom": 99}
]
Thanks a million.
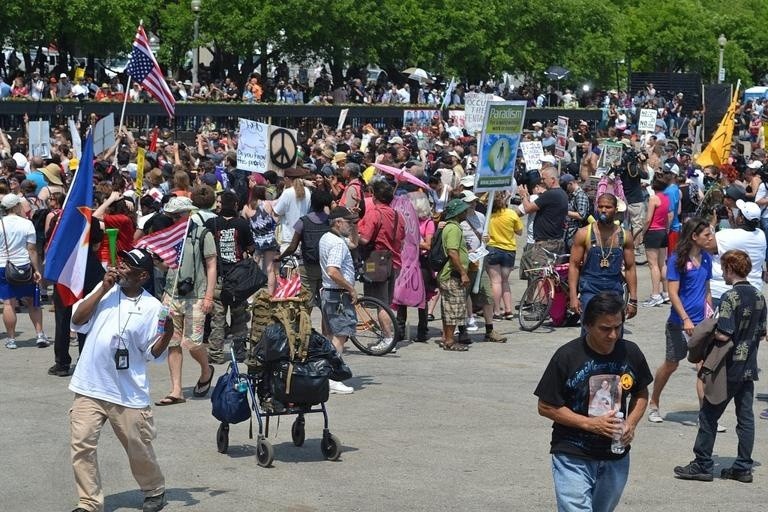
[
  {"left": 211, "top": 291, "right": 351, "bottom": 425},
  {"left": 222, "top": 259, "right": 267, "bottom": 302},
  {"left": 5, "top": 260, "right": 33, "bottom": 285},
  {"left": 364, "top": 251, "right": 392, "bottom": 282}
]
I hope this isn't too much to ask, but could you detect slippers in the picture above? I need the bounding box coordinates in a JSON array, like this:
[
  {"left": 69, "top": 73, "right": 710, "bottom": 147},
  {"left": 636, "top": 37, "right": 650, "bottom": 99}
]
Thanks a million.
[
  {"left": 193, "top": 365, "right": 214, "bottom": 397},
  {"left": 155, "top": 396, "right": 186, "bottom": 405}
]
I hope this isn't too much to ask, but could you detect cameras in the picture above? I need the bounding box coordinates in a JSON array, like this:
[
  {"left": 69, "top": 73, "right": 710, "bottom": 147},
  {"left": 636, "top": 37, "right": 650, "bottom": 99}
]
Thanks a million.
[
  {"left": 710, "top": 204, "right": 729, "bottom": 219},
  {"left": 177, "top": 276, "right": 195, "bottom": 296},
  {"left": 641, "top": 81, "right": 650, "bottom": 91},
  {"left": 178, "top": 143, "right": 186, "bottom": 150}
]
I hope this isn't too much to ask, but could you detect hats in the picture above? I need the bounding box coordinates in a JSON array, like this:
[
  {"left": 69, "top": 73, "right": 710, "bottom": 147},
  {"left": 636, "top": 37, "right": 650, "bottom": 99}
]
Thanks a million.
[
  {"left": 540, "top": 155, "right": 574, "bottom": 185},
  {"left": 532, "top": 121, "right": 542, "bottom": 128},
  {"left": 145, "top": 164, "right": 172, "bottom": 184},
  {"left": 664, "top": 144, "right": 691, "bottom": 175},
  {"left": 1, "top": 193, "right": 20, "bottom": 209},
  {"left": 328, "top": 206, "right": 358, "bottom": 219},
  {"left": 284, "top": 167, "right": 299, "bottom": 179},
  {"left": 199, "top": 173, "right": 217, "bottom": 185},
  {"left": 13, "top": 152, "right": 63, "bottom": 189},
  {"left": 388, "top": 137, "right": 403, "bottom": 144},
  {"left": 736, "top": 199, "right": 761, "bottom": 222},
  {"left": 119, "top": 249, "right": 153, "bottom": 269},
  {"left": 164, "top": 196, "right": 199, "bottom": 213},
  {"left": 322, "top": 150, "right": 346, "bottom": 163},
  {"left": 445, "top": 175, "right": 479, "bottom": 220},
  {"left": 747, "top": 148, "right": 765, "bottom": 168}
]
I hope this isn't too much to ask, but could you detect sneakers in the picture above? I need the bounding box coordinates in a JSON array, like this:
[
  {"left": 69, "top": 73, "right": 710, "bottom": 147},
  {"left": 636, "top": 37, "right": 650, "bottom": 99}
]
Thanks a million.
[
  {"left": 642, "top": 293, "right": 670, "bottom": 307},
  {"left": 5, "top": 338, "right": 17, "bottom": 348},
  {"left": 440, "top": 312, "right": 513, "bottom": 351},
  {"left": 648, "top": 408, "right": 663, "bottom": 422},
  {"left": 721, "top": 468, "right": 753, "bottom": 482},
  {"left": 143, "top": 493, "right": 164, "bottom": 512},
  {"left": 371, "top": 338, "right": 396, "bottom": 352},
  {"left": 48, "top": 364, "right": 69, "bottom": 376},
  {"left": 717, "top": 425, "right": 726, "bottom": 431},
  {"left": 36, "top": 332, "right": 50, "bottom": 347},
  {"left": 674, "top": 464, "right": 713, "bottom": 481}
]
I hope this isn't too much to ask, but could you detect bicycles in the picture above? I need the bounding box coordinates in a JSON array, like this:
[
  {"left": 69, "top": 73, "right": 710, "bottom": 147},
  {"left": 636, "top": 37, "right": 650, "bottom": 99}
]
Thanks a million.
[
  {"left": 273, "top": 252, "right": 398, "bottom": 355},
  {"left": 517, "top": 245, "right": 628, "bottom": 330}
]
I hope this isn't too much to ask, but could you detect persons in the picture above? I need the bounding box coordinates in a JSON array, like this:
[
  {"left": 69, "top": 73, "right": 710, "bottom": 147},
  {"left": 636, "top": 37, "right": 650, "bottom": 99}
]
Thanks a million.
[
  {"left": 673, "top": 248, "right": 768, "bottom": 484},
  {"left": 531, "top": 291, "right": 655, "bottom": 512},
  {"left": 64, "top": 246, "right": 176, "bottom": 511},
  {"left": 1, "top": 46, "right": 768, "bottom": 435}
]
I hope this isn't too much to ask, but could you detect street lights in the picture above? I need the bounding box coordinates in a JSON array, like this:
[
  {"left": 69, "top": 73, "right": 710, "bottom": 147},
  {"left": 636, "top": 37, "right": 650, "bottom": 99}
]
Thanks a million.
[
  {"left": 716, "top": 32, "right": 728, "bottom": 86},
  {"left": 190, "top": 1, "right": 201, "bottom": 88}
]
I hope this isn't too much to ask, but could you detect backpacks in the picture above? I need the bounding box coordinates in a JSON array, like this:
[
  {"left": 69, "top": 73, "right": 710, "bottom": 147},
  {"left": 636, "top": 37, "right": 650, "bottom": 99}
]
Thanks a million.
[
  {"left": 300, "top": 215, "right": 331, "bottom": 263},
  {"left": 428, "top": 222, "right": 453, "bottom": 271}
]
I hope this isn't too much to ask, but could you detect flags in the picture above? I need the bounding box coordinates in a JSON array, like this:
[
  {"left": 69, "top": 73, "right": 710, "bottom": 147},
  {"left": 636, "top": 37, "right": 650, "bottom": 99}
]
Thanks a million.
[{"left": 122, "top": 24, "right": 178, "bottom": 121}]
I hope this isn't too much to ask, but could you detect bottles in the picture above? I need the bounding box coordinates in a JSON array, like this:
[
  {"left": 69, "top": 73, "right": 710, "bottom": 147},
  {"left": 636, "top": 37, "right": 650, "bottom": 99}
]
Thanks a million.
[
  {"left": 33, "top": 283, "right": 40, "bottom": 308},
  {"left": 609, "top": 411, "right": 626, "bottom": 457},
  {"left": 157, "top": 306, "right": 170, "bottom": 335}
]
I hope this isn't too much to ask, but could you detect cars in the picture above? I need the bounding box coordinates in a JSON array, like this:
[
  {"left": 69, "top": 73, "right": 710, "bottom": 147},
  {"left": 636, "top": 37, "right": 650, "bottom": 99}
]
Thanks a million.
[
  {"left": 366, "top": 69, "right": 386, "bottom": 85},
  {"left": 4, "top": 48, "right": 126, "bottom": 76}
]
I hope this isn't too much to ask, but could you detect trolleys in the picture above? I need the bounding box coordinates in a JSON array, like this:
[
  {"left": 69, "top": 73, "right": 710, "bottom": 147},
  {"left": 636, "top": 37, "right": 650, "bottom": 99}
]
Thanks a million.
[{"left": 215, "top": 287, "right": 351, "bottom": 467}]
